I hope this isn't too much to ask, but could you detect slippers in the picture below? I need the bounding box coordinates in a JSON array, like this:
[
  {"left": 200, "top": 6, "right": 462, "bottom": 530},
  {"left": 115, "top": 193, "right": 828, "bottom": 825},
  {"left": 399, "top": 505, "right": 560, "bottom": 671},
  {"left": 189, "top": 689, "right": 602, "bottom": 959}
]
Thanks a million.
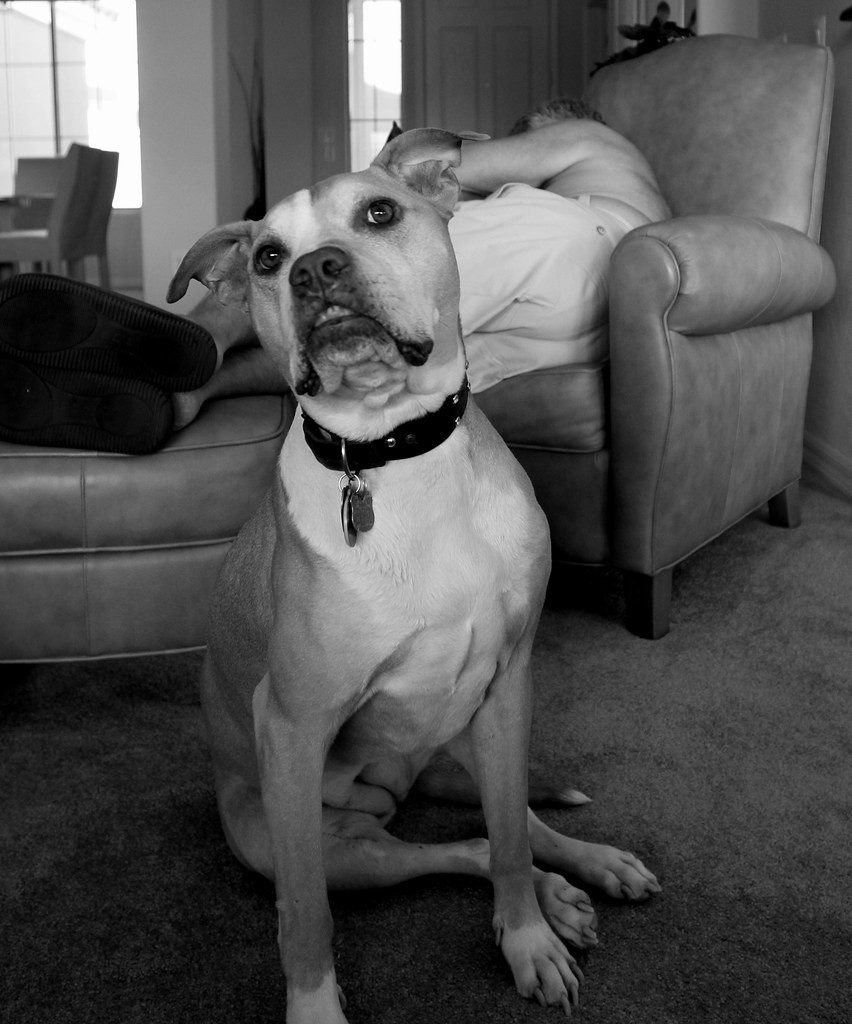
[{"left": 0, "top": 273, "right": 218, "bottom": 455}]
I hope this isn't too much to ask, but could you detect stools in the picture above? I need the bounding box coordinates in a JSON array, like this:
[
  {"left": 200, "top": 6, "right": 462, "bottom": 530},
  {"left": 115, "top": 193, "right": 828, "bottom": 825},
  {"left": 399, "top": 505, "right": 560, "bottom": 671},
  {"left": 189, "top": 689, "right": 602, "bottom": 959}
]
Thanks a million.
[{"left": 0, "top": 389, "right": 294, "bottom": 729}]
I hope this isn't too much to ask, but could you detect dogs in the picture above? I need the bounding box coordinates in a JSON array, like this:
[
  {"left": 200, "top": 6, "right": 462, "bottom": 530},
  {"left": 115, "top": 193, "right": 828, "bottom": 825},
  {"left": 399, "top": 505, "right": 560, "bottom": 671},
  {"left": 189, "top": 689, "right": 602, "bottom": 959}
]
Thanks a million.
[{"left": 166, "top": 122, "right": 661, "bottom": 1024}]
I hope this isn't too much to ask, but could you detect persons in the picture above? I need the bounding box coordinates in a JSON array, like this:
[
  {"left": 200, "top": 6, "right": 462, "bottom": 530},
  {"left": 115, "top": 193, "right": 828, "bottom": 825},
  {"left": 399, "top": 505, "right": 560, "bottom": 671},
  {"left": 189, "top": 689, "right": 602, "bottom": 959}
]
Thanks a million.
[{"left": 0, "top": 96, "right": 673, "bottom": 456}]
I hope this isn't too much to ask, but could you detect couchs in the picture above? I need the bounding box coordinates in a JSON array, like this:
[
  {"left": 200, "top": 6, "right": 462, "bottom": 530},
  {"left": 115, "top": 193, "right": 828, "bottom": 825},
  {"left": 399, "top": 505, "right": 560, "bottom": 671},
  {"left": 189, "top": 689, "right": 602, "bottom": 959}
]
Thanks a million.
[{"left": 471, "top": 34, "right": 836, "bottom": 641}]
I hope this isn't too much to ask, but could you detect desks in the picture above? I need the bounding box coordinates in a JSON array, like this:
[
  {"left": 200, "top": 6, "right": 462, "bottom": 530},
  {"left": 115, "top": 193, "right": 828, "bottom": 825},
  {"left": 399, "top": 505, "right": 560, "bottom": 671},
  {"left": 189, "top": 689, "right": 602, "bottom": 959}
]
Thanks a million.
[{"left": 0, "top": 192, "right": 53, "bottom": 283}]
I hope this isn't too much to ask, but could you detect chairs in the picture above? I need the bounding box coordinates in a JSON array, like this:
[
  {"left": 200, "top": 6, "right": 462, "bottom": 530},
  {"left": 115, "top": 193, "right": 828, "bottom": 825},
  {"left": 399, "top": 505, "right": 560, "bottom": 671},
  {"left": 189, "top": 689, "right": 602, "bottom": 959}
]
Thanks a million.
[{"left": 0, "top": 144, "right": 117, "bottom": 290}]
